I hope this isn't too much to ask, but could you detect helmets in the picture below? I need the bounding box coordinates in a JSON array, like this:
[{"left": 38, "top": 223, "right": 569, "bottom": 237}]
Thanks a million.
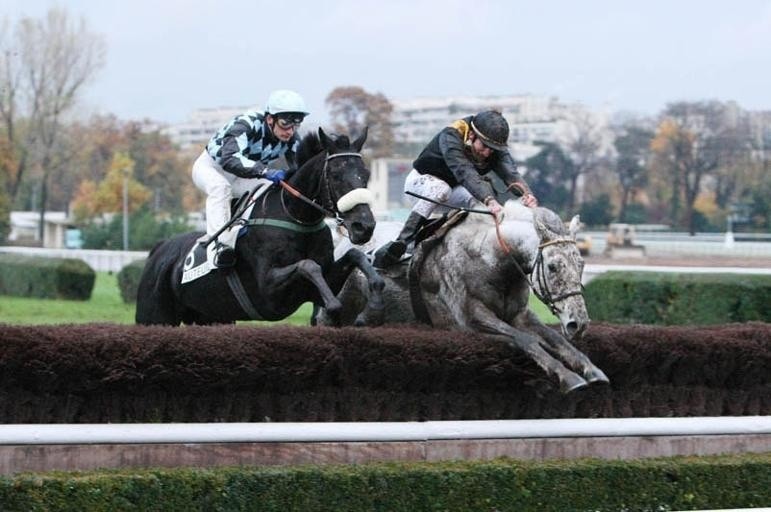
[
  {"left": 470, "top": 109, "right": 512, "bottom": 155},
  {"left": 266, "top": 90, "right": 311, "bottom": 116}
]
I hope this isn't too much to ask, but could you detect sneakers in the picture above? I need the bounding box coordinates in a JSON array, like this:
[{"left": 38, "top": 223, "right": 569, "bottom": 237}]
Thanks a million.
[{"left": 216, "top": 247, "right": 236, "bottom": 277}]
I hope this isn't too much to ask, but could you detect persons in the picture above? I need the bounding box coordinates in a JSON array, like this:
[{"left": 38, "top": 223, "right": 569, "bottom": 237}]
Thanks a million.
[
  {"left": 376, "top": 108, "right": 539, "bottom": 267},
  {"left": 568, "top": 214, "right": 580, "bottom": 242},
  {"left": 607, "top": 226, "right": 633, "bottom": 246},
  {"left": 191, "top": 88, "right": 311, "bottom": 270}
]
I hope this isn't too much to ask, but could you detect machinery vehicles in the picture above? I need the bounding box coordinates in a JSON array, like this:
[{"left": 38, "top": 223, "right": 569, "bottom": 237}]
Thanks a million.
[
  {"left": 605, "top": 221, "right": 646, "bottom": 259},
  {"left": 564, "top": 222, "right": 592, "bottom": 255}
]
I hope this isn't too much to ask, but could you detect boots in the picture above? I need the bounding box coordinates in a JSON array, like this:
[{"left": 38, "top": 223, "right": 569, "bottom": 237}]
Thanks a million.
[{"left": 381, "top": 211, "right": 428, "bottom": 267}]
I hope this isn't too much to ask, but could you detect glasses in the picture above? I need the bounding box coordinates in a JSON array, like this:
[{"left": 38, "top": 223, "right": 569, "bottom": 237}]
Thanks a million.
[{"left": 276, "top": 115, "right": 303, "bottom": 129}]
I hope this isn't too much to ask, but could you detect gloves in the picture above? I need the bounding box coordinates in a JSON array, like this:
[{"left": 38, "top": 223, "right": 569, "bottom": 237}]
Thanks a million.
[{"left": 261, "top": 167, "right": 286, "bottom": 187}]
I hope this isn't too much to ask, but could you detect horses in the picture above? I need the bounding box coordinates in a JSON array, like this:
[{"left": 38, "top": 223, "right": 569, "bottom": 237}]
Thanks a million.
[
  {"left": 134, "top": 125, "right": 387, "bottom": 328},
  {"left": 311, "top": 194, "right": 615, "bottom": 398}
]
